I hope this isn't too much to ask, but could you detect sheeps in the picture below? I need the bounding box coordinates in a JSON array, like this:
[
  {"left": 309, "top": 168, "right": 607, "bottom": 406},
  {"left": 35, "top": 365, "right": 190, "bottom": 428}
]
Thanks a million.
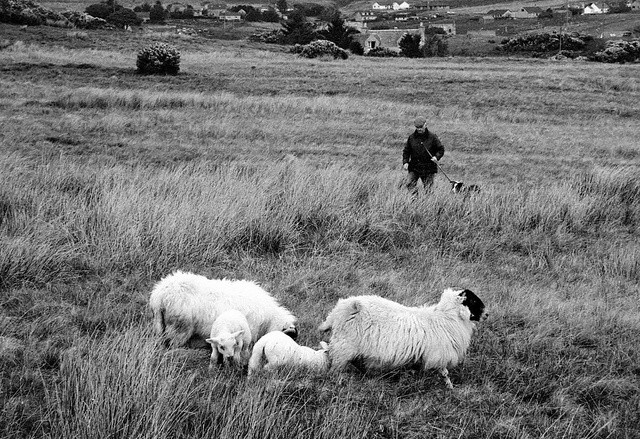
[
  {"left": 318, "top": 286, "right": 490, "bottom": 392},
  {"left": 206, "top": 309, "right": 252, "bottom": 372},
  {"left": 247, "top": 330, "right": 330, "bottom": 381},
  {"left": 149, "top": 270, "right": 299, "bottom": 353}
]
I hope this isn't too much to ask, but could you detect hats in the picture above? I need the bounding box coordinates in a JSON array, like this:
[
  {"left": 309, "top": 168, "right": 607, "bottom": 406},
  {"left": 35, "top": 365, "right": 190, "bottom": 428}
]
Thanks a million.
[{"left": 414, "top": 118, "right": 426, "bottom": 128}]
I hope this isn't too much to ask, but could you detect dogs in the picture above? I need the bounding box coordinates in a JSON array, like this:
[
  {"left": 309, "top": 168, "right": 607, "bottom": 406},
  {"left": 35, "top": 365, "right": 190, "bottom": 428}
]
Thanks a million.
[{"left": 450, "top": 180, "right": 481, "bottom": 195}]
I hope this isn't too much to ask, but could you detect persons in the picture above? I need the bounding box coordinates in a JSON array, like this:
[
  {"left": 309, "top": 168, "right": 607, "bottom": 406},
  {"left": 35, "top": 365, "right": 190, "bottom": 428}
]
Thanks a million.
[{"left": 402, "top": 118, "right": 445, "bottom": 200}]
